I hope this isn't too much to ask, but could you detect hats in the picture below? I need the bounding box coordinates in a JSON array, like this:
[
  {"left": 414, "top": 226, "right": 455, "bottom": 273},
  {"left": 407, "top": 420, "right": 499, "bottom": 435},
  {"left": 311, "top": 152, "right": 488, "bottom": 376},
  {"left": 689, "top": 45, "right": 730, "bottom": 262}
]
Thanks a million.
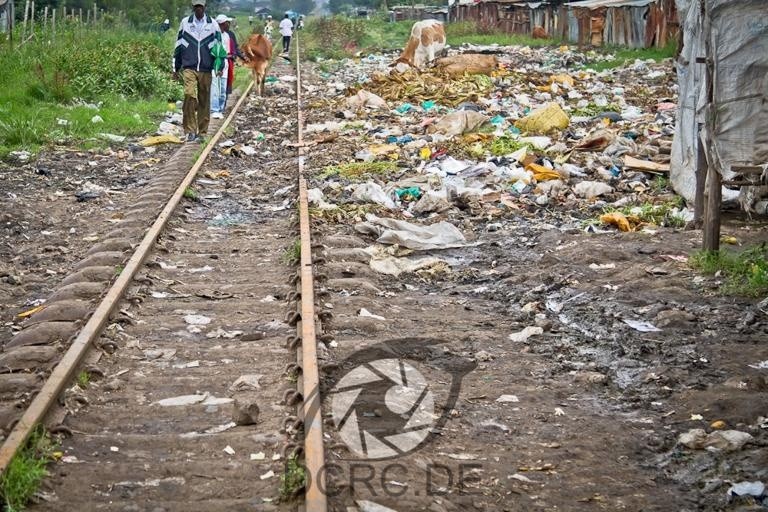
[
  {"left": 215, "top": 14, "right": 233, "bottom": 24},
  {"left": 191, "top": 0, "right": 206, "bottom": 7}
]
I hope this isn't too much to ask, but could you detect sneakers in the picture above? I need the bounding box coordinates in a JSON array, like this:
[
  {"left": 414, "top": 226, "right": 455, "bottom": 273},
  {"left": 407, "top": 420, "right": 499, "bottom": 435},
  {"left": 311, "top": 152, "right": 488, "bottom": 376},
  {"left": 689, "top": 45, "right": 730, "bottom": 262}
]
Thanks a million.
[
  {"left": 212, "top": 112, "right": 224, "bottom": 119},
  {"left": 186, "top": 132, "right": 206, "bottom": 142}
]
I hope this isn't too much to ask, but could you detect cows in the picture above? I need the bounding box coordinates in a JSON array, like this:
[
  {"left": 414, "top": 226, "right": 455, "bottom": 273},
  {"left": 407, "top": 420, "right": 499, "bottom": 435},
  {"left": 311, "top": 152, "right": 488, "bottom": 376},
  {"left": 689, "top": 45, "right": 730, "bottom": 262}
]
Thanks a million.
[
  {"left": 233, "top": 32, "right": 293, "bottom": 98},
  {"left": 387, "top": 19, "right": 448, "bottom": 73}
]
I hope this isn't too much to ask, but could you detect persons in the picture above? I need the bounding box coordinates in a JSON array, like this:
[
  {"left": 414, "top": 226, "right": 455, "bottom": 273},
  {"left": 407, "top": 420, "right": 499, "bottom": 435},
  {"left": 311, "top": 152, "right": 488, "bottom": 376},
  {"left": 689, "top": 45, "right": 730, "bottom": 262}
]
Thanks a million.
[
  {"left": 159, "top": 17, "right": 171, "bottom": 33},
  {"left": 170, "top": 0, "right": 225, "bottom": 144},
  {"left": 211, "top": 14, "right": 250, "bottom": 120},
  {"left": 290, "top": 15, "right": 296, "bottom": 30},
  {"left": 279, "top": 14, "right": 294, "bottom": 53},
  {"left": 297, "top": 15, "right": 303, "bottom": 29},
  {"left": 262, "top": 14, "right": 275, "bottom": 40},
  {"left": 248, "top": 13, "right": 253, "bottom": 24}
]
